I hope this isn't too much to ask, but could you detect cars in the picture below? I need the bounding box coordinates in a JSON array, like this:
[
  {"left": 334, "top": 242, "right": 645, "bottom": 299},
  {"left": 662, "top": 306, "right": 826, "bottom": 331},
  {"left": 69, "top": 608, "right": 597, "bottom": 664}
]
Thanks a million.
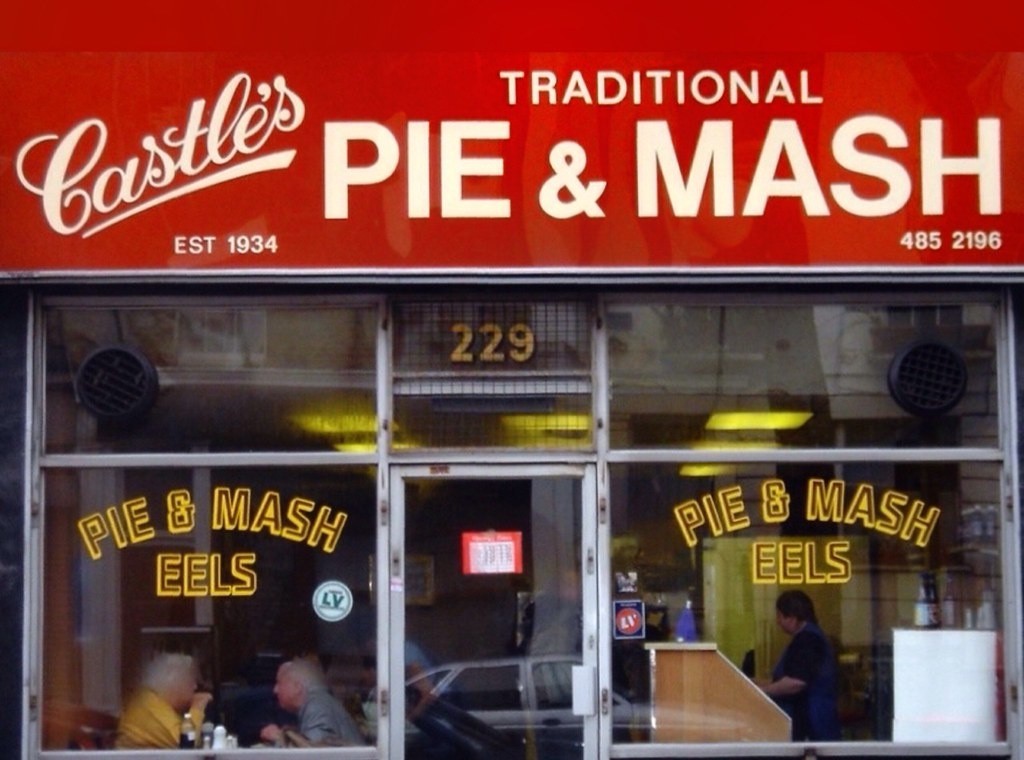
[{"left": 368, "top": 653, "right": 765, "bottom": 760}]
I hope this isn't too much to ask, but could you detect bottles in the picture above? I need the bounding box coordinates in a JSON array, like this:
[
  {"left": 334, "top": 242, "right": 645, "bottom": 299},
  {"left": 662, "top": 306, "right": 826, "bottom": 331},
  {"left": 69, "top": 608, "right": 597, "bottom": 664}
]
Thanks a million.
[
  {"left": 941, "top": 577, "right": 998, "bottom": 629},
  {"left": 958, "top": 504, "right": 997, "bottom": 546},
  {"left": 178, "top": 713, "right": 195, "bottom": 748},
  {"left": 912, "top": 568, "right": 939, "bottom": 629},
  {"left": 200, "top": 722, "right": 214, "bottom": 749}
]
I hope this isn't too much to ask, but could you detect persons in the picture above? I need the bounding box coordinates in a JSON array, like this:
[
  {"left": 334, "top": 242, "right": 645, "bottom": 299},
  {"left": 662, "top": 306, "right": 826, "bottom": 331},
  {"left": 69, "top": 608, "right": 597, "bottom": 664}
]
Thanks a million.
[
  {"left": 224, "top": 648, "right": 334, "bottom": 749},
  {"left": 109, "top": 652, "right": 212, "bottom": 750},
  {"left": 350, "top": 606, "right": 458, "bottom": 725},
  {"left": 756, "top": 589, "right": 842, "bottom": 744},
  {"left": 260, "top": 660, "right": 366, "bottom": 748},
  {"left": 514, "top": 592, "right": 647, "bottom": 760}
]
